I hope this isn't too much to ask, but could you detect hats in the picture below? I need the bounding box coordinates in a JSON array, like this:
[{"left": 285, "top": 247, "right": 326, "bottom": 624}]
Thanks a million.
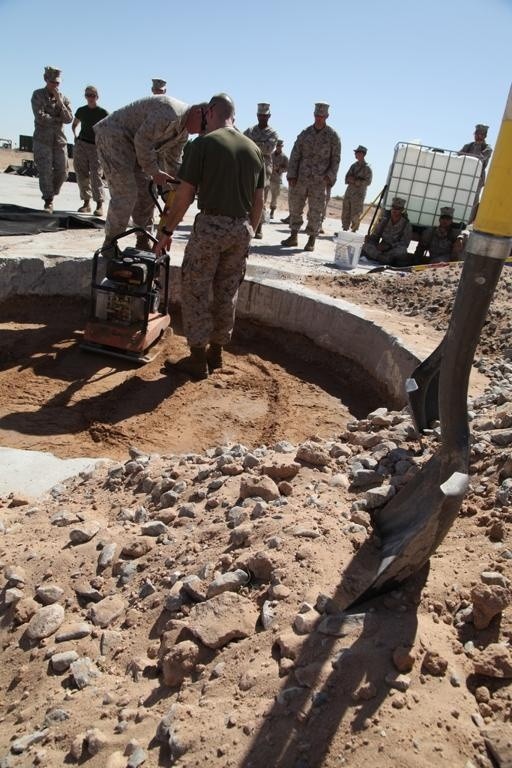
[
  {"left": 152, "top": 79, "right": 166, "bottom": 90},
  {"left": 314, "top": 103, "right": 328, "bottom": 117},
  {"left": 257, "top": 103, "right": 270, "bottom": 115},
  {"left": 353, "top": 145, "right": 367, "bottom": 152},
  {"left": 392, "top": 197, "right": 406, "bottom": 207},
  {"left": 476, "top": 124, "right": 489, "bottom": 133},
  {"left": 441, "top": 207, "right": 454, "bottom": 216},
  {"left": 276, "top": 140, "right": 284, "bottom": 147},
  {"left": 44, "top": 65, "right": 61, "bottom": 82}
]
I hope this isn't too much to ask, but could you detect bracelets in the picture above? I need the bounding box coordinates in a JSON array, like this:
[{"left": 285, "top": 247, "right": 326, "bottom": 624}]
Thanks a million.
[{"left": 161, "top": 225, "right": 173, "bottom": 237}]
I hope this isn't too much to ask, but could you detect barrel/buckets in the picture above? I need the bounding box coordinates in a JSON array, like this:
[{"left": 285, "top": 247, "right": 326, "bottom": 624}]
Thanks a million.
[{"left": 334, "top": 231, "right": 366, "bottom": 269}]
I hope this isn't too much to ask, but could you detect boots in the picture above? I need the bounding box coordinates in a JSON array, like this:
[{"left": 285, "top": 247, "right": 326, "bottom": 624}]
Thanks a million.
[
  {"left": 205, "top": 343, "right": 223, "bottom": 373},
  {"left": 78, "top": 200, "right": 91, "bottom": 212},
  {"left": 93, "top": 201, "right": 103, "bottom": 216},
  {"left": 256, "top": 208, "right": 325, "bottom": 250},
  {"left": 137, "top": 235, "right": 152, "bottom": 252},
  {"left": 377, "top": 254, "right": 393, "bottom": 264},
  {"left": 100, "top": 235, "right": 121, "bottom": 259},
  {"left": 44, "top": 198, "right": 53, "bottom": 211},
  {"left": 164, "top": 347, "right": 209, "bottom": 378}
]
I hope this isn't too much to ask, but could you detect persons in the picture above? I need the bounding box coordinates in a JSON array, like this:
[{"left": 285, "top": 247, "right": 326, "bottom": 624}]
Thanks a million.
[
  {"left": 459, "top": 124, "right": 493, "bottom": 224},
  {"left": 151, "top": 80, "right": 167, "bottom": 97},
  {"left": 411, "top": 207, "right": 470, "bottom": 265},
  {"left": 155, "top": 93, "right": 265, "bottom": 381},
  {"left": 341, "top": 145, "right": 372, "bottom": 232},
  {"left": 72, "top": 86, "right": 109, "bottom": 217},
  {"left": 244, "top": 100, "right": 342, "bottom": 252},
  {"left": 365, "top": 197, "right": 413, "bottom": 265},
  {"left": 31, "top": 66, "right": 73, "bottom": 215},
  {"left": 92, "top": 95, "right": 209, "bottom": 259}
]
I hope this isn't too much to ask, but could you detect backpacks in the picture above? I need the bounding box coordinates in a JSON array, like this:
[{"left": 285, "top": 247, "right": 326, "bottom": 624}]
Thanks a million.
[{"left": 385, "top": 210, "right": 409, "bottom": 236}]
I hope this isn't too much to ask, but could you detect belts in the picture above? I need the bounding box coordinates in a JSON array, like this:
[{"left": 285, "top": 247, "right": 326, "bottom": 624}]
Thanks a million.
[
  {"left": 201, "top": 207, "right": 249, "bottom": 218},
  {"left": 77, "top": 136, "right": 95, "bottom": 144}
]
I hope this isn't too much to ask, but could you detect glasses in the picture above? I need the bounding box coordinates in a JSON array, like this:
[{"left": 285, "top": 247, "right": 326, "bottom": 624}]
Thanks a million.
[
  {"left": 84, "top": 92, "right": 97, "bottom": 97},
  {"left": 440, "top": 215, "right": 452, "bottom": 220},
  {"left": 200, "top": 103, "right": 215, "bottom": 132}
]
